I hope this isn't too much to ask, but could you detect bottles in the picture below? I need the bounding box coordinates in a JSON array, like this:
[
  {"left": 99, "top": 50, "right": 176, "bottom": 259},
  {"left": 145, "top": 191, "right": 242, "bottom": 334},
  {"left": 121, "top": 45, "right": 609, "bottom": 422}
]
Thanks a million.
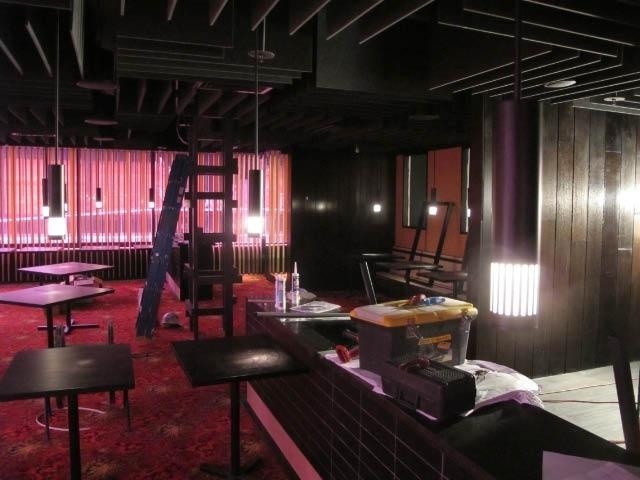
[{"left": 291, "top": 261, "right": 302, "bottom": 307}]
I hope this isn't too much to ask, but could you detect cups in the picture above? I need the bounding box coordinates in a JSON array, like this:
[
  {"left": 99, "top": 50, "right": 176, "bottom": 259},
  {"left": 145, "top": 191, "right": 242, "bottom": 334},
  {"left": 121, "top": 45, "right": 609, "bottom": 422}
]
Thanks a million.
[{"left": 274, "top": 272, "right": 287, "bottom": 310}]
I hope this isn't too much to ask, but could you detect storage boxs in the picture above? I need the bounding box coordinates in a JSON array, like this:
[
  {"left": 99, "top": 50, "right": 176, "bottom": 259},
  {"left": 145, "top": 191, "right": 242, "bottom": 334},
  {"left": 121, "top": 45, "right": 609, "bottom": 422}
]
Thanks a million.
[{"left": 349, "top": 295, "right": 480, "bottom": 377}]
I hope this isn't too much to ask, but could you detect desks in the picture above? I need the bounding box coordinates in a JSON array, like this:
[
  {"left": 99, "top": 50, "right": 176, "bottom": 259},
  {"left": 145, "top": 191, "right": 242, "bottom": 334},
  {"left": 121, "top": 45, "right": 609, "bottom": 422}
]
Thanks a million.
[
  {"left": 1, "top": 282, "right": 116, "bottom": 433},
  {"left": 16, "top": 260, "right": 115, "bottom": 334},
  {"left": 165, "top": 331, "right": 307, "bottom": 479},
  {"left": 2, "top": 342, "right": 136, "bottom": 479}
]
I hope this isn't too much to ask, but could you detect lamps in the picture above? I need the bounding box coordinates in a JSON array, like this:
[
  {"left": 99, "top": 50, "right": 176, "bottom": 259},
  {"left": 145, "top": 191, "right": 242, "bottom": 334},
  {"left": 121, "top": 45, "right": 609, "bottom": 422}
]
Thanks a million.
[
  {"left": 248, "top": 31, "right": 261, "bottom": 234},
  {"left": 148, "top": 187, "right": 154, "bottom": 209},
  {"left": 39, "top": 10, "right": 64, "bottom": 241},
  {"left": 95, "top": 139, "right": 102, "bottom": 209},
  {"left": 372, "top": 156, "right": 382, "bottom": 212},
  {"left": 425, "top": 152, "right": 438, "bottom": 216}
]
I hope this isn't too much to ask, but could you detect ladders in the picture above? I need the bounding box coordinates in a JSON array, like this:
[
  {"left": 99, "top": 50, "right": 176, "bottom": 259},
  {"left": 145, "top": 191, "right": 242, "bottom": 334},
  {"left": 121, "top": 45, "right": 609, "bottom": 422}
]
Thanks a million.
[{"left": 136, "top": 154, "right": 191, "bottom": 338}]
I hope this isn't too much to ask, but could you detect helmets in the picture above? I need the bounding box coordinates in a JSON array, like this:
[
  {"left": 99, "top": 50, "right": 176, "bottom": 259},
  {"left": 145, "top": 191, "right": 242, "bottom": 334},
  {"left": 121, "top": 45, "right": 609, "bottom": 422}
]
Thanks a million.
[{"left": 161, "top": 311, "right": 185, "bottom": 329}]
